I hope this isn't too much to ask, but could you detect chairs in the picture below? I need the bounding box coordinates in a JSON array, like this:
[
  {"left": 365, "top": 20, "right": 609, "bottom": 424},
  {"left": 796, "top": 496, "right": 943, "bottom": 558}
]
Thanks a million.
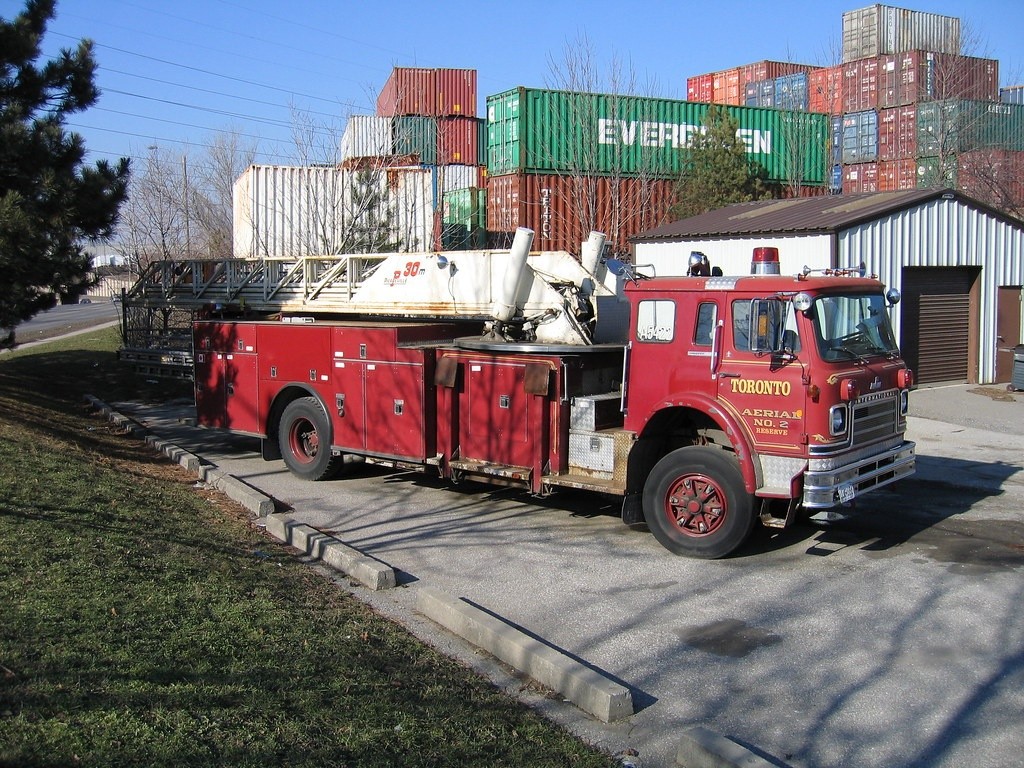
[{"left": 781, "top": 329, "right": 801, "bottom": 353}]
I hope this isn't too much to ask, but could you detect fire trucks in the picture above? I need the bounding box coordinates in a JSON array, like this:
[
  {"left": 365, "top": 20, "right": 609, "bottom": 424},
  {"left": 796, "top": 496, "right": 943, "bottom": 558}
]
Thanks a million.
[{"left": 114, "top": 226, "right": 918, "bottom": 559}]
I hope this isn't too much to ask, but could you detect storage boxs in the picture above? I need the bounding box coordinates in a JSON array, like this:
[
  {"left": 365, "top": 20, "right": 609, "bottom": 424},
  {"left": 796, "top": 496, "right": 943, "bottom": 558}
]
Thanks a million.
[
  {"left": 233, "top": 2, "right": 1024, "bottom": 263},
  {"left": 569, "top": 391, "right": 625, "bottom": 431}
]
